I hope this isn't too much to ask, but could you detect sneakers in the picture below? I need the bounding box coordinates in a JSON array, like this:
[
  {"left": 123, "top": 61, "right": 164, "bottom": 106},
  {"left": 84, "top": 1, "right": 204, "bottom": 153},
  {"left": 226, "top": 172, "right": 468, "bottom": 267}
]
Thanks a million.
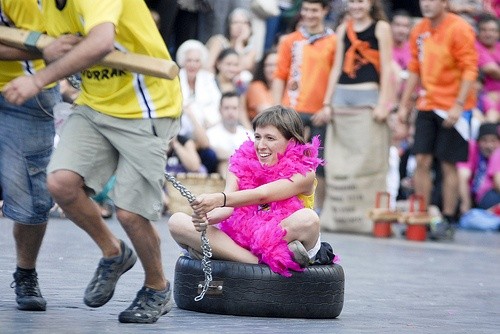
[
  {"left": 82, "top": 239, "right": 137, "bottom": 308},
  {"left": 10, "top": 271, "right": 47, "bottom": 311},
  {"left": 119, "top": 279, "right": 174, "bottom": 324}
]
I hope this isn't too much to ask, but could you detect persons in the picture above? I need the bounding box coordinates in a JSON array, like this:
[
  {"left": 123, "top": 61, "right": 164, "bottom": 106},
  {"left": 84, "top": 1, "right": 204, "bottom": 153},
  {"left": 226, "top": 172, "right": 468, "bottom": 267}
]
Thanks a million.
[
  {"left": 48, "top": 0, "right": 500, "bottom": 241},
  {"left": 0, "top": 0, "right": 185, "bottom": 324},
  {"left": 0, "top": 0, "right": 63, "bottom": 314},
  {"left": 169, "top": 104, "right": 321, "bottom": 268}
]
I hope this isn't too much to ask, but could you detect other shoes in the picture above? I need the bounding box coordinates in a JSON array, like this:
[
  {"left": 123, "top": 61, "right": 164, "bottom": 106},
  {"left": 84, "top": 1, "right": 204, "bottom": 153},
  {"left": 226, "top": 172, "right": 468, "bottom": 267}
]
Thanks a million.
[
  {"left": 188, "top": 246, "right": 209, "bottom": 260},
  {"left": 401, "top": 222, "right": 456, "bottom": 240},
  {"left": 287, "top": 239, "right": 310, "bottom": 268}
]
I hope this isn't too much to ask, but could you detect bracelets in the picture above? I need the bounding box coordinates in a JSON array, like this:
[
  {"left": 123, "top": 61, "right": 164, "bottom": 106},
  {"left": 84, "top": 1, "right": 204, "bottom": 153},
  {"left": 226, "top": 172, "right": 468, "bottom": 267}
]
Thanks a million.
[
  {"left": 220, "top": 192, "right": 226, "bottom": 208},
  {"left": 455, "top": 100, "right": 464, "bottom": 106}
]
